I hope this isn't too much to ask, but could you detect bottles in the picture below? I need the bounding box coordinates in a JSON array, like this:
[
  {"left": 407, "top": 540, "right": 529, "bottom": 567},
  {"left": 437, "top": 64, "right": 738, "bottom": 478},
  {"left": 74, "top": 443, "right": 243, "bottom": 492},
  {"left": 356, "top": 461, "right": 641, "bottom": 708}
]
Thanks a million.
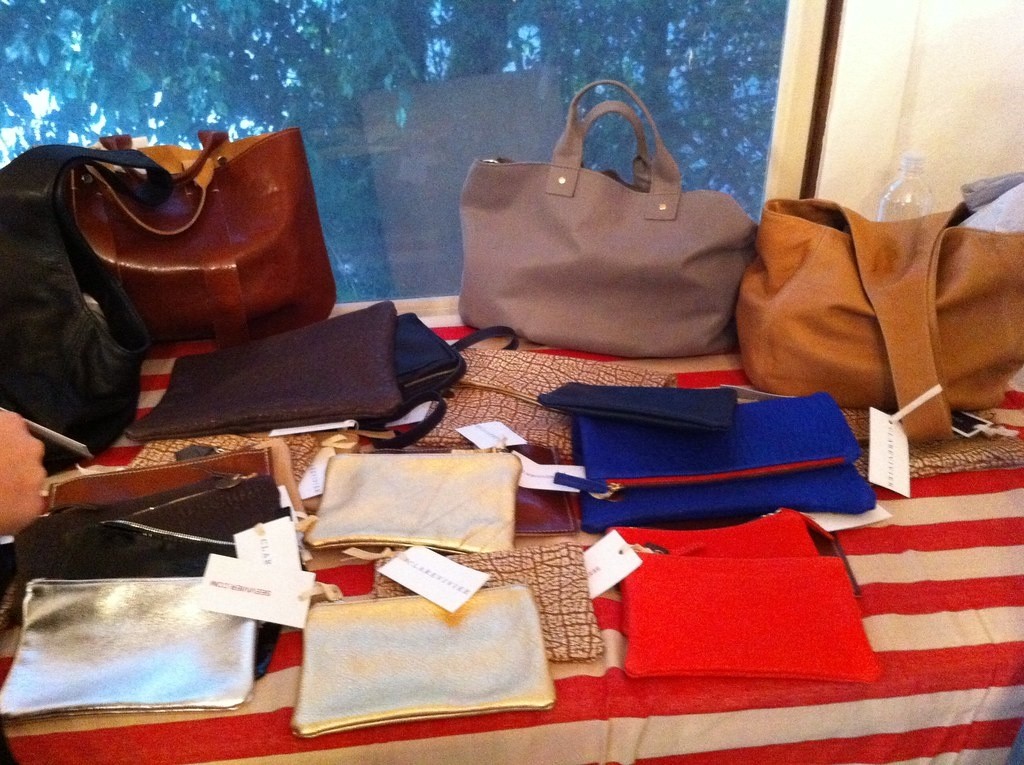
[{"left": 876, "top": 156, "right": 931, "bottom": 221}]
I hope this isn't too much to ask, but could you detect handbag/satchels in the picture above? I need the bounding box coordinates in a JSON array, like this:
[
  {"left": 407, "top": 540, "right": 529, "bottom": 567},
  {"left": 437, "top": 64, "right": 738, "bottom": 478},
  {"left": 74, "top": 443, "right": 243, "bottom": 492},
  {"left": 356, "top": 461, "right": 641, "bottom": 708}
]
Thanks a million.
[
  {"left": 538, "top": 380, "right": 732, "bottom": 430},
  {"left": 69, "top": 127, "right": 336, "bottom": 340},
  {"left": 735, "top": 197, "right": 1024, "bottom": 440},
  {"left": 0, "top": 301, "right": 881, "bottom": 739},
  {"left": 458, "top": 78, "right": 758, "bottom": 359},
  {"left": 0, "top": 145, "right": 175, "bottom": 470}
]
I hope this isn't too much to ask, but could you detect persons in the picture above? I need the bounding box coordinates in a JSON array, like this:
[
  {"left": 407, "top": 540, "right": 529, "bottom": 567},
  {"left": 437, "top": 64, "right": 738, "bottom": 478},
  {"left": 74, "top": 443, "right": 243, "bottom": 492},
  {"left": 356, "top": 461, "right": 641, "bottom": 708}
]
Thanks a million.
[{"left": 0, "top": 412, "right": 48, "bottom": 533}]
